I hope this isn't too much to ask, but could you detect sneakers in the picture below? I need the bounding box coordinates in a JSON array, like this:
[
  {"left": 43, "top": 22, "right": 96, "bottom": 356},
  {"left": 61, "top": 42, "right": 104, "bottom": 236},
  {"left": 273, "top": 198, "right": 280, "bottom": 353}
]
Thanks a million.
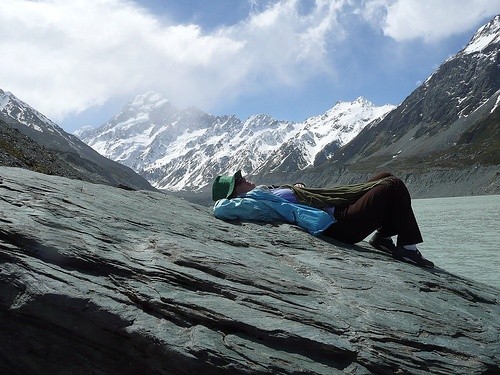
[
  {"left": 393, "top": 246, "right": 434, "bottom": 268},
  {"left": 369, "top": 232, "right": 395, "bottom": 254}
]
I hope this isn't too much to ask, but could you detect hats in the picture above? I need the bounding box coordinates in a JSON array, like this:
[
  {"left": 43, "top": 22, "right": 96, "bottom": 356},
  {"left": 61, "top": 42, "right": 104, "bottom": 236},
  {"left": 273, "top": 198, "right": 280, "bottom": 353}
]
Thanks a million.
[{"left": 212, "top": 169, "right": 241, "bottom": 202}]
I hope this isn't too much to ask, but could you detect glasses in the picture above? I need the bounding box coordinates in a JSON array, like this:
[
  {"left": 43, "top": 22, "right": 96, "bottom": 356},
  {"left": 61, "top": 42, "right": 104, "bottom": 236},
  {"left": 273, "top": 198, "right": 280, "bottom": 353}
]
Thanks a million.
[{"left": 234, "top": 176, "right": 242, "bottom": 189}]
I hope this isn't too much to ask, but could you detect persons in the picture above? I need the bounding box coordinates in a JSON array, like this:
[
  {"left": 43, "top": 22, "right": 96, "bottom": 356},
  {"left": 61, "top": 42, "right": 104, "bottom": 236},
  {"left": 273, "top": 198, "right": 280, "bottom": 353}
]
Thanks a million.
[{"left": 213, "top": 169, "right": 436, "bottom": 268}]
